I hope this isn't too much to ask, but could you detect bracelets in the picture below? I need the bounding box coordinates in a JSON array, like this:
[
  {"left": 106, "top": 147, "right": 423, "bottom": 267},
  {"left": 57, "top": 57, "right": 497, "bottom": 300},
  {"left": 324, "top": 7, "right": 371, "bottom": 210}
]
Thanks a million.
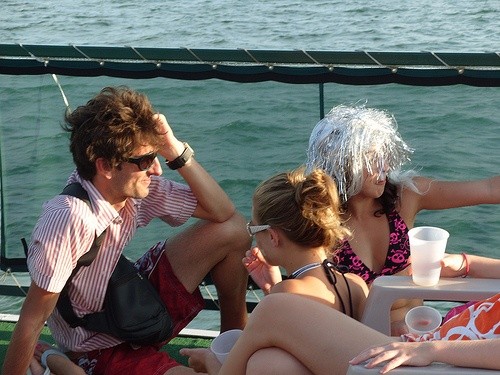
[{"left": 461, "top": 253, "right": 469, "bottom": 278}]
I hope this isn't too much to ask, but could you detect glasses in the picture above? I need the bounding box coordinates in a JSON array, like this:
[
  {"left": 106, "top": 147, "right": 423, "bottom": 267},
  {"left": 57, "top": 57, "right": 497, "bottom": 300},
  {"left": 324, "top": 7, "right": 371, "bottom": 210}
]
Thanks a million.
[
  {"left": 125, "top": 148, "right": 163, "bottom": 171},
  {"left": 247, "top": 218, "right": 271, "bottom": 237}
]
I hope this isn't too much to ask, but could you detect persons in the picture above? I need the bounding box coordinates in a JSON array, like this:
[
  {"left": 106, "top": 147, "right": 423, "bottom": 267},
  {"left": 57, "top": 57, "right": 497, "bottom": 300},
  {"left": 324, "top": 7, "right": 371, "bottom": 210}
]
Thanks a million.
[
  {"left": 2, "top": 86, "right": 254, "bottom": 375},
  {"left": 306, "top": 106, "right": 500, "bottom": 337},
  {"left": 26, "top": 166, "right": 369, "bottom": 375},
  {"left": 219, "top": 253, "right": 500, "bottom": 375}
]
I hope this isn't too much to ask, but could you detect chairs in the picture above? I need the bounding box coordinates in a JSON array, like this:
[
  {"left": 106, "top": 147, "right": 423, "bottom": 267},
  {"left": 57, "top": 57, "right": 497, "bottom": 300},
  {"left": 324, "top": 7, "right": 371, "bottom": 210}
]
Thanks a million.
[{"left": 346, "top": 275, "right": 500, "bottom": 375}]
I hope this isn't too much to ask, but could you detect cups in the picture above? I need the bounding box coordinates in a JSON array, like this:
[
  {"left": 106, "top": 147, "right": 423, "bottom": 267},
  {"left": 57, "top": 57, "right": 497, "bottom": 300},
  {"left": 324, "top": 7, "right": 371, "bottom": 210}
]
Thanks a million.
[
  {"left": 405, "top": 306, "right": 442, "bottom": 334},
  {"left": 210, "top": 329, "right": 243, "bottom": 365},
  {"left": 408, "top": 226, "right": 449, "bottom": 286}
]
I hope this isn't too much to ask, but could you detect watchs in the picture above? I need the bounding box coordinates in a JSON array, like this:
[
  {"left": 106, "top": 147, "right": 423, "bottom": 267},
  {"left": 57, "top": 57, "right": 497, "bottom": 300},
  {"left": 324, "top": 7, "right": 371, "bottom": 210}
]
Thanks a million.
[{"left": 165, "top": 142, "right": 194, "bottom": 170}]
